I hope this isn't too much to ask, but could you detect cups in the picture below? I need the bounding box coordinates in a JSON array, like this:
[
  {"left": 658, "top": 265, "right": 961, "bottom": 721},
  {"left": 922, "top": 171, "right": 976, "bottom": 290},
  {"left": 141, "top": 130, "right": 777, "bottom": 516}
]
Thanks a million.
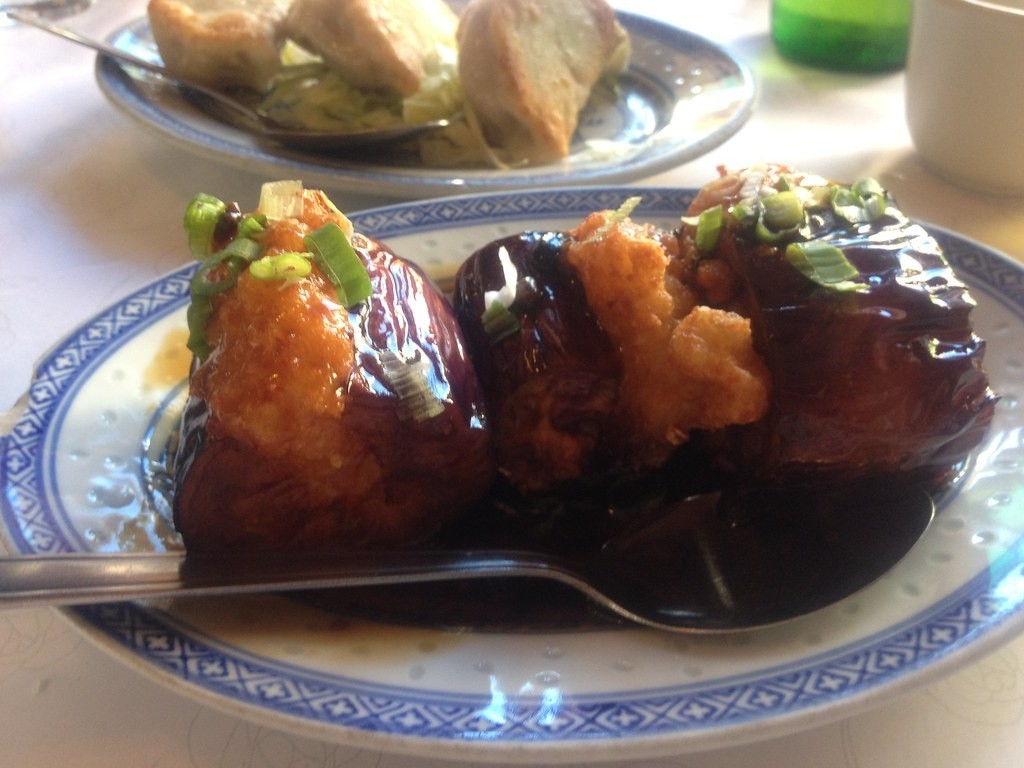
[
  {"left": 768, "top": 0, "right": 909, "bottom": 78},
  {"left": 907, "top": 0, "right": 1024, "bottom": 194}
]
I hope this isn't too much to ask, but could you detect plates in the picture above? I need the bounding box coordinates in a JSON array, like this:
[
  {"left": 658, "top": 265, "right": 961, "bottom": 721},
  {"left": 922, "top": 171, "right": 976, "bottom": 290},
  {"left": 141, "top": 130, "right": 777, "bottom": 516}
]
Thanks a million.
[
  {"left": 0, "top": 183, "right": 1021, "bottom": 768},
  {"left": 94, "top": 0, "right": 759, "bottom": 204}
]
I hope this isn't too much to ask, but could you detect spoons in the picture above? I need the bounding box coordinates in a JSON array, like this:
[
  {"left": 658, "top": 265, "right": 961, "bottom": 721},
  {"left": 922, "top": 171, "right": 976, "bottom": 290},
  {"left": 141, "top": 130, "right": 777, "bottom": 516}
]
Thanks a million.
[
  {"left": 3, "top": 10, "right": 451, "bottom": 155},
  {"left": 0, "top": 475, "right": 939, "bottom": 638}
]
